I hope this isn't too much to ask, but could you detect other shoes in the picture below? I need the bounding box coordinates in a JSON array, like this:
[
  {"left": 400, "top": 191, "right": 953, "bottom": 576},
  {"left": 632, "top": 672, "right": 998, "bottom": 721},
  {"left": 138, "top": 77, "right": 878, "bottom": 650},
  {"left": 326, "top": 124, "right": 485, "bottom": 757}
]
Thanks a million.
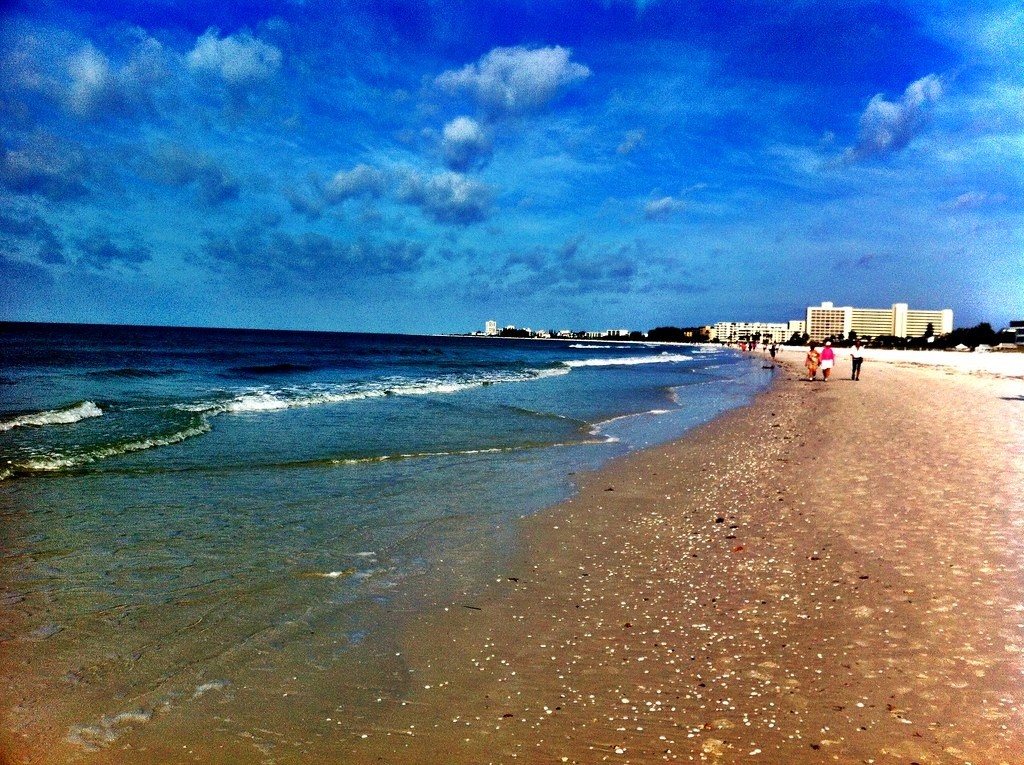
[
  {"left": 852, "top": 375, "right": 855, "bottom": 380},
  {"left": 813, "top": 376, "right": 816, "bottom": 379},
  {"left": 856, "top": 377, "right": 859, "bottom": 380},
  {"left": 809, "top": 377, "right": 813, "bottom": 380},
  {"left": 825, "top": 378, "right": 828, "bottom": 381}
]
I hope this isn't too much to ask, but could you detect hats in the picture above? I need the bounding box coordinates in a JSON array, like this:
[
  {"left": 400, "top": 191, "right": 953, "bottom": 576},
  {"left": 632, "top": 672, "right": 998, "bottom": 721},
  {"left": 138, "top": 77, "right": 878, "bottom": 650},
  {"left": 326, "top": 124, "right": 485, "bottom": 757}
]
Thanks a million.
[{"left": 826, "top": 342, "right": 831, "bottom": 345}]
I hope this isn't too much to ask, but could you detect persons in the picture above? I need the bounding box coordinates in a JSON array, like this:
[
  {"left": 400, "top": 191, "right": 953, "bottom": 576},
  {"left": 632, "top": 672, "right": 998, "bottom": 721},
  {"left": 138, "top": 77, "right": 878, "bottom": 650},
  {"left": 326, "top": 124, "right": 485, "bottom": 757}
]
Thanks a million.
[
  {"left": 727, "top": 340, "right": 785, "bottom": 360},
  {"left": 850, "top": 339, "right": 865, "bottom": 381},
  {"left": 804, "top": 342, "right": 821, "bottom": 381},
  {"left": 820, "top": 340, "right": 836, "bottom": 381}
]
